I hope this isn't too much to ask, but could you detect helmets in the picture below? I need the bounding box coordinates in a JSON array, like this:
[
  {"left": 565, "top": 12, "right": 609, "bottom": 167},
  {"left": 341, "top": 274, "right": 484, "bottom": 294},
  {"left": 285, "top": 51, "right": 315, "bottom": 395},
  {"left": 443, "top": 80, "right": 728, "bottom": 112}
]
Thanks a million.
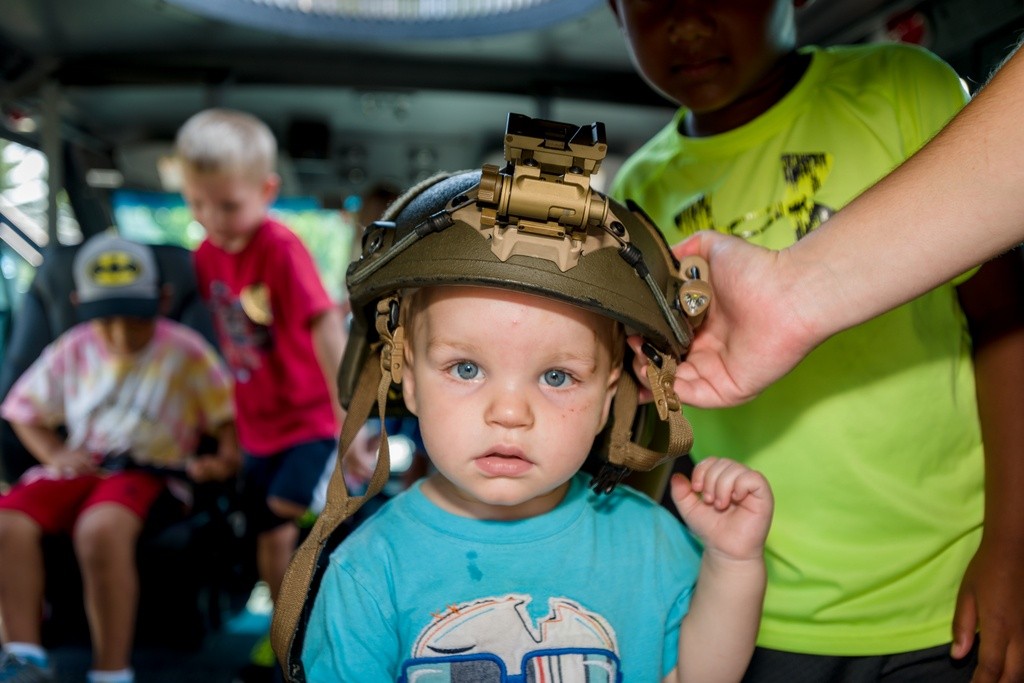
[{"left": 345, "top": 169, "right": 694, "bottom": 367}]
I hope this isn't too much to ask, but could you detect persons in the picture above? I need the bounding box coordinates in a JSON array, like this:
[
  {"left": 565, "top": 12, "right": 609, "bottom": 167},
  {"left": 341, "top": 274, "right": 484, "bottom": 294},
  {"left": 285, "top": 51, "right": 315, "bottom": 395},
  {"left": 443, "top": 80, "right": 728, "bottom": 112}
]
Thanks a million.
[
  {"left": 0, "top": 237, "right": 242, "bottom": 683},
  {"left": 607, "top": 0, "right": 1024, "bottom": 683},
  {"left": 627, "top": 43, "right": 1024, "bottom": 409},
  {"left": 270, "top": 113, "right": 773, "bottom": 682},
  {"left": 181, "top": 111, "right": 347, "bottom": 664}
]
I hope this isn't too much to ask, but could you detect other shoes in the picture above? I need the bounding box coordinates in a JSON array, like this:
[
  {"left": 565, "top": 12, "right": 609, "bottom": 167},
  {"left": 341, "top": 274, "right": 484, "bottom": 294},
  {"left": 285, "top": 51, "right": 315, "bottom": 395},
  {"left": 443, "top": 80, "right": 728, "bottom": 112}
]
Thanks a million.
[
  {"left": 249, "top": 635, "right": 276, "bottom": 666},
  {"left": 0, "top": 650, "right": 52, "bottom": 683}
]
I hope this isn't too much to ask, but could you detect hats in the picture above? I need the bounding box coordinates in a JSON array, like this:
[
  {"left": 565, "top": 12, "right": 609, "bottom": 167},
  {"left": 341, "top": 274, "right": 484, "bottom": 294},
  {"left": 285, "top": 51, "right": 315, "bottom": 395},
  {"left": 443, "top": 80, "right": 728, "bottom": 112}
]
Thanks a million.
[{"left": 72, "top": 231, "right": 161, "bottom": 320}]
